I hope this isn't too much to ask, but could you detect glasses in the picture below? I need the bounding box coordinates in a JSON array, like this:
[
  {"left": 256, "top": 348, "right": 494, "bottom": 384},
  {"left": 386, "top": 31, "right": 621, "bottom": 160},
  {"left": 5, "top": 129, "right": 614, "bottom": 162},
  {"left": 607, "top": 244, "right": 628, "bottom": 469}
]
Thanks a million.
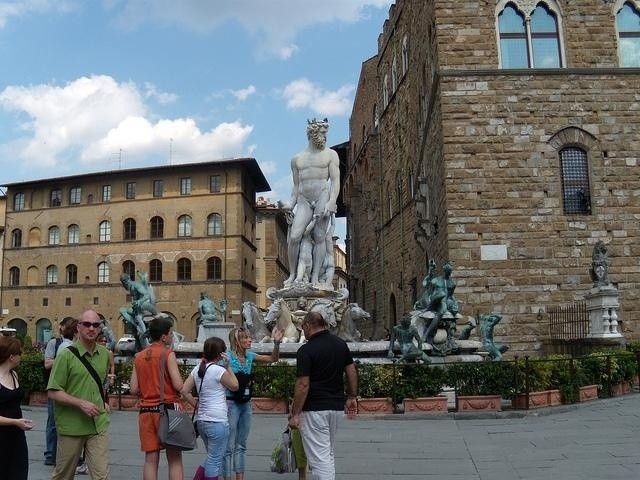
[
  {"left": 301, "top": 323, "right": 309, "bottom": 329},
  {"left": 79, "top": 321, "right": 101, "bottom": 328},
  {"left": 234, "top": 327, "right": 248, "bottom": 342}
]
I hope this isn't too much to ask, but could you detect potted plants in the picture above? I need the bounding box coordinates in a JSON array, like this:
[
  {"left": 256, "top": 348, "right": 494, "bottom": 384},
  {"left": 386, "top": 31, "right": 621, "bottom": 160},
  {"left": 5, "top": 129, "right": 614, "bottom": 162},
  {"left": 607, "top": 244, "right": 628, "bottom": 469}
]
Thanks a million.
[
  {"left": 576, "top": 361, "right": 598, "bottom": 403},
  {"left": 632, "top": 356, "right": 640, "bottom": 385},
  {"left": 352, "top": 361, "right": 397, "bottom": 414},
  {"left": 511, "top": 355, "right": 548, "bottom": 409},
  {"left": 399, "top": 361, "right": 448, "bottom": 413},
  {"left": 106, "top": 363, "right": 142, "bottom": 411},
  {"left": 608, "top": 356, "right": 620, "bottom": 397},
  {"left": 248, "top": 362, "right": 292, "bottom": 415},
  {"left": 177, "top": 364, "right": 213, "bottom": 414},
  {"left": 548, "top": 359, "right": 573, "bottom": 405},
  {"left": 622, "top": 357, "right": 631, "bottom": 393},
  {"left": 449, "top": 355, "right": 519, "bottom": 412},
  {"left": 21, "top": 356, "right": 51, "bottom": 408}
]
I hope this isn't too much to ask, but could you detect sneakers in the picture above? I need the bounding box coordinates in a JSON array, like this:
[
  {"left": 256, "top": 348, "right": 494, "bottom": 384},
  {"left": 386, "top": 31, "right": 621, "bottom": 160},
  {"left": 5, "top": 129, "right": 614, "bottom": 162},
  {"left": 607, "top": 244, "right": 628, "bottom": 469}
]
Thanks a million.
[{"left": 74, "top": 465, "right": 88, "bottom": 475}]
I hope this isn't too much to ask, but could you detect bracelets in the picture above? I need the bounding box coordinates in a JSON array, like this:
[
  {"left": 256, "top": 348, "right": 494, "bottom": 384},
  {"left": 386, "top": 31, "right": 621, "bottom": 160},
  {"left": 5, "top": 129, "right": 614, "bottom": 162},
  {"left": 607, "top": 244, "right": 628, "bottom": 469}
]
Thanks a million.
[
  {"left": 273, "top": 339, "right": 282, "bottom": 344},
  {"left": 349, "top": 394, "right": 357, "bottom": 399}
]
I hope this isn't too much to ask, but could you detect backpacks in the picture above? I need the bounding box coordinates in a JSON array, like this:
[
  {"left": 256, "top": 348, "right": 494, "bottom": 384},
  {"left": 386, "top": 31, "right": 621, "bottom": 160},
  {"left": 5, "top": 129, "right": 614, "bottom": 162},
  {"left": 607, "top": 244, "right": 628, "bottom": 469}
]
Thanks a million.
[
  {"left": 269, "top": 425, "right": 297, "bottom": 474},
  {"left": 230, "top": 371, "right": 254, "bottom": 405}
]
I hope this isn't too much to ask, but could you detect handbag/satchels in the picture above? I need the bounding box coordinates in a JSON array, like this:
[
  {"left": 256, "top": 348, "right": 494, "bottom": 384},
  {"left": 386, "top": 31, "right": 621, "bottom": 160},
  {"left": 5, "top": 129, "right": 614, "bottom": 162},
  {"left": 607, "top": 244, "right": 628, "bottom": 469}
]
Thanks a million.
[
  {"left": 193, "top": 421, "right": 201, "bottom": 439},
  {"left": 158, "top": 350, "right": 196, "bottom": 451}
]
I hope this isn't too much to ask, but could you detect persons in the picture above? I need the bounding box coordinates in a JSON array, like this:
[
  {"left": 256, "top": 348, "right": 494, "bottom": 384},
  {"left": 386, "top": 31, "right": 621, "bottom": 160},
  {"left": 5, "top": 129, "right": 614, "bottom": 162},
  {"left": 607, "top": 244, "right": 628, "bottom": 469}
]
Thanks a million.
[
  {"left": 195, "top": 291, "right": 222, "bottom": 344},
  {"left": 184, "top": 337, "right": 240, "bottom": 480},
  {"left": 118, "top": 273, "right": 159, "bottom": 337},
  {"left": 478, "top": 312, "right": 504, "bottom": 361},
  {"left": 415, "top": 259, "right": 440, "bottom": 311},
  {"left": 218, "top": 326, "right": 285, "bottom": 480},
  {"left": 44, "top": 317, "right": 83, "bottom": 465},
  {"left": 422, "top": 259, "right": 460, "bottom": 341},
  {"left": 46, "top": 310, "right": 112, "bottom": 480},
  {"left": 98, "top": 334, "right": 114, "bottom": 397},
  {"left": 288, "top": 392, "right": 311, "bottom": 480},
  {"left": 54, "top": 319, "right": 90, "bottom": 475},
  {"left": 0, "top": 336, "right": 35, "bottom": 480},
  {"left": 281, "top": 117, "right": 341, "bottom": 288},
  {"left": 131, "top": 320, "right": 199, "bottom": 480},
  {"left": 99, "top": 320, "right": 115, "bottom": 352},
  {"left": 289, "top": 312, "right": 358, "bottom": 480},
  {"left": 388, "top": 315, "right": 432, "bottom": 366}
]
